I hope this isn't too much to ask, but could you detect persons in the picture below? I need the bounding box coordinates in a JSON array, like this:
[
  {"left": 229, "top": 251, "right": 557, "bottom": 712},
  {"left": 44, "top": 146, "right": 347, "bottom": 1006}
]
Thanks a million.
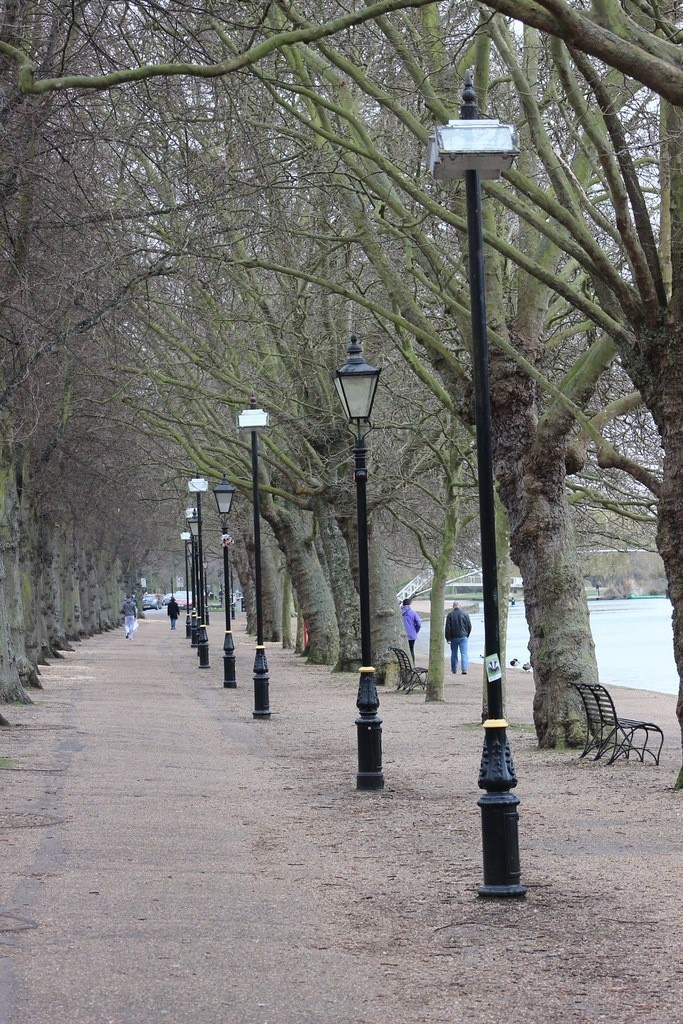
[
  {"left": 119, "top": 594, "right": 139, "bottom": 641},
  {"left": 167, "top": 597, "right": 180, "bottom": 631},
  {"left": 445, "top": 600, "right": 473, "bottom": 675},
  {"left": 400, "top": 599, "right": 422, "bottom": 666},
  {"left": 510, "top": 597, "right": 516, "bottom": 607}
]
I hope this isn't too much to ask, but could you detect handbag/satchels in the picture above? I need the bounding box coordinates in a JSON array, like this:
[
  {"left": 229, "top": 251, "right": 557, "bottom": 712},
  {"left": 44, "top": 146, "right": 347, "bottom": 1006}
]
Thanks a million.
[{"left": 133, "top": 620, "right": 139, "bottom": 632}]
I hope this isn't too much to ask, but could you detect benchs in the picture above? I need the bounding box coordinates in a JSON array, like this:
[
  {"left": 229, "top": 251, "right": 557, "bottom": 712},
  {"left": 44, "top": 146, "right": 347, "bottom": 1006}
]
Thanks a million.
[
  {"left": 566, "top": 681, "right": 664, "bottom": 765},
  {"left": 387, "top": 646, "right": 429, "bottom": 695}
]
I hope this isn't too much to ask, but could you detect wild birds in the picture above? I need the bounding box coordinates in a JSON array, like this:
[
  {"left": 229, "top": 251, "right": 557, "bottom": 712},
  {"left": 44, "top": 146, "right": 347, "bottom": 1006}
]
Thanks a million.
[
  {"left": 522, "top": 662, "right": 532, "bottom": 672},
  {"left": 510, "top": 659, "right": 521, "bottom": 668}
]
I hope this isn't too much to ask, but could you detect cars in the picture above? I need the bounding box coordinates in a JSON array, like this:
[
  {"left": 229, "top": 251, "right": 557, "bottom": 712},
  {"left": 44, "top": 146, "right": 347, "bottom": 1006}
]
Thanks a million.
[{"left": 142, "top": 591, "right": 197, "bottom": 610}]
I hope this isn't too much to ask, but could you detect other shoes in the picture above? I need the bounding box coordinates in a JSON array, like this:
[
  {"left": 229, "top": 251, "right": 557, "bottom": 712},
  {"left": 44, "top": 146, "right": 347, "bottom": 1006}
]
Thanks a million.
[
  {"left": 462, "top": 672, "right": 467, "bottom": 674},
  {"left": 453, "top": 672, "right": 456, "bottom": 674},
  {"left": 171, "top": 627, "right": 175, "bottom": 630},
  {"left": 129, "top": 637, "right": 134, "bottom": 641},
  {"left": 126, "top": 632, "right": 129, "bottom": 638}
]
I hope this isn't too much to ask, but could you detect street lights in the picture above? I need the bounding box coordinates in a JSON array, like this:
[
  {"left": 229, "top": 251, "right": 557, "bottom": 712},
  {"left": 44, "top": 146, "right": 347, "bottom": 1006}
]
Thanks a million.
[
  {"left": 234, "top": 395, "right": 272, "bottom": 718},
  {"left": 330, "top": 332, "right": 384, "bottom": 791},
  {"left": 212, "top": 472, "right": 238, "bottom": 688},
  {"left": 426, "top": 63, "right": 530, "bottom": 897},
  {"left": 180, "top": 467, "right": 210, "bottom": 672}
]
